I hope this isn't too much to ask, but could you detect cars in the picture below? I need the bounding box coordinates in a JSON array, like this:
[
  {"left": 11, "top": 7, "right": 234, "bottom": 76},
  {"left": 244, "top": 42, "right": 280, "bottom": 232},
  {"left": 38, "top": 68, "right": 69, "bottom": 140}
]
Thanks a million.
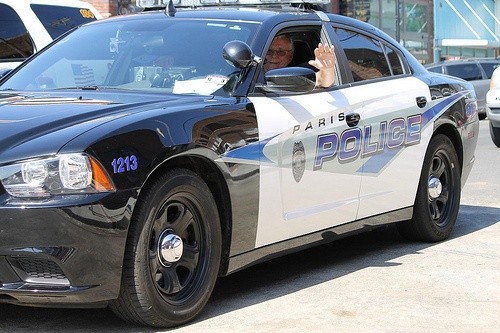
[
  {"left": 1, "top": 7, "right": 479, "bottom": 327},
  {"left": 486, "top": 63, "right": 500, "bottom": 148}
]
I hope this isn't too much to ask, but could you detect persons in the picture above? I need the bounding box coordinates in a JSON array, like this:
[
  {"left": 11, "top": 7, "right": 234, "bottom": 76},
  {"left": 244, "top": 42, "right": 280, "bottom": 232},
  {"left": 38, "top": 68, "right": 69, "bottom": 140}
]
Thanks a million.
[
  {"left": 262, "top": 33, "right": 336, "bottom": 88},
  {"left": 347, "top": 47, "right": 388, "bottom": 82}
]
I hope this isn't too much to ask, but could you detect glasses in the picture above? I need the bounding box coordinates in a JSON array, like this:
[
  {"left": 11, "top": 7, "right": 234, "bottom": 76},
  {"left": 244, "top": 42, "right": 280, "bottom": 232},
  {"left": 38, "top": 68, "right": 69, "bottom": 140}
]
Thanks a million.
[{"left": 266, "top": 49, "right": 291, "bottom": 56}]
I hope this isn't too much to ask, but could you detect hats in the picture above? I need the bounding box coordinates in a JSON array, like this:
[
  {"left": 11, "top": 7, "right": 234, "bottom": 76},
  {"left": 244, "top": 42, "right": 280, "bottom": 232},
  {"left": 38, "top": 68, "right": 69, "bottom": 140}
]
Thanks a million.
[{"left": 133, "top": 34, "right": 174, "bottom": 63}]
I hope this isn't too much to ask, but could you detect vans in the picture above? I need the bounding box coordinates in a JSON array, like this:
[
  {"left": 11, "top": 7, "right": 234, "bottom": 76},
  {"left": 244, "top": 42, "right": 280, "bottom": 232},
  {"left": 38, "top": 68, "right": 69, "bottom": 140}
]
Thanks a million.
[
  {"left": 0, "top": 1, "right": 125, "bottom": 90},
  {"left": 424, "top": 59, "right": 496, "bottom": 118}
]
